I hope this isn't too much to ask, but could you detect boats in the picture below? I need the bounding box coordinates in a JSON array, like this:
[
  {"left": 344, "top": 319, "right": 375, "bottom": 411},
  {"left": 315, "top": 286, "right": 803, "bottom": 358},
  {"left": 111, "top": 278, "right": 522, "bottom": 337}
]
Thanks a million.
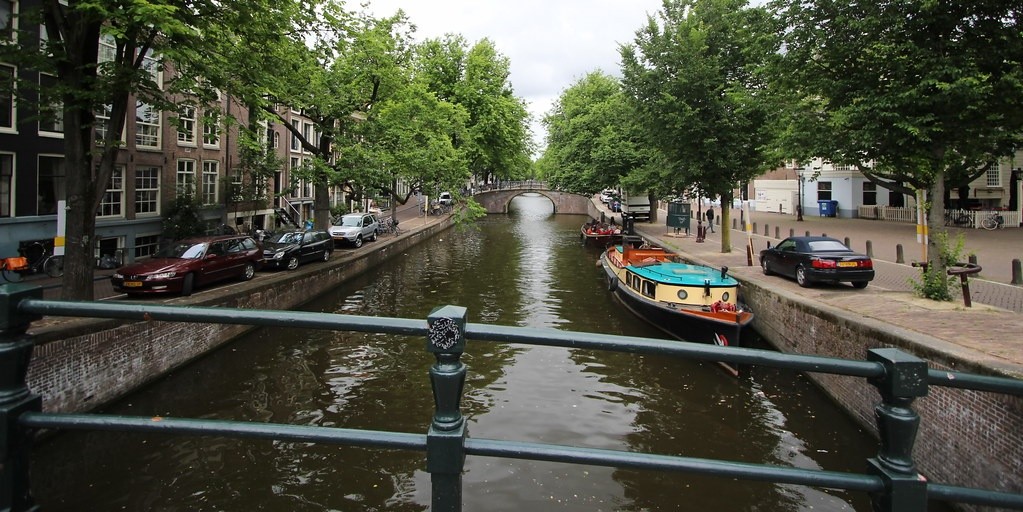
[
  {"left": 596, "top": 237, "right": 754, "bottom": 378},
  {"left": 581, "top": 219, "right": 622, "bottom": 249}
]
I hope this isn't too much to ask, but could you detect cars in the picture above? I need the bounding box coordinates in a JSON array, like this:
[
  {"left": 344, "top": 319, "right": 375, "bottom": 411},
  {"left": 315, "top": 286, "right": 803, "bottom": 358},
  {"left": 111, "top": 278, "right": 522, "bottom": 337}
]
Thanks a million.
[
  {"left": 759, "top": 236, "right": 875, "bottom": 290},
  {"left": 608, "top": 198, "right": 621, "bottom": 212},
  {"left": 327, "top": 213, "right": 379, "bottom": 248},
  {"left": 111, "top": 236, "right": 265, "bottom": 299},
  {"left": 258, "top": 229, "right": 334, "bottom": 271},
  {"left": 439, "top": 192, "right": 452, "bottom": 205},
  {"left": 599, "top": 189, "right": 618, "bottom": 204},
  {"left": 460, "top": 178, "right": 515, "bottom": 199}
]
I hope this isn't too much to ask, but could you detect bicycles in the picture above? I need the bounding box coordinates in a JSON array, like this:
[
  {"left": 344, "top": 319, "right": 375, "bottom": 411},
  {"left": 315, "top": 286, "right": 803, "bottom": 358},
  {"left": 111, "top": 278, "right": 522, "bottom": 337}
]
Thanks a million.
[
  {"left": 43, "top": 237, "right": 102, "bottom": 278},
  {"left": 420, "top": 201, "right": 453, "bottom": 217},
  {"left": 378, "top": 216, "right": 402, "bottom": 238},
  {"left": 981, "top": 211, "right": 1005, "bottom": 230},
  {"left": 2, "top": 240, "right": 54, "bottom": 283},
  {"left": 945, "top": 208, "right": 973, "bottom": 229}
]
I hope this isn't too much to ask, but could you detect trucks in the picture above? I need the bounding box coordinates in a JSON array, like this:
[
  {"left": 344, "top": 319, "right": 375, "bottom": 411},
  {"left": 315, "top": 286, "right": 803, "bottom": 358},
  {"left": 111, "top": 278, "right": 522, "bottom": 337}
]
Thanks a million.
[{"left": 618, "top": 185, "right": 650, "bottom": 222}]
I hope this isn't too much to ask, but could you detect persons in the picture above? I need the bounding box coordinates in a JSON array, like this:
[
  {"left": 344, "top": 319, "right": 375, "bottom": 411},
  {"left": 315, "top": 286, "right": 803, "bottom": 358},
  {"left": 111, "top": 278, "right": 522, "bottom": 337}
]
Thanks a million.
[
  {"left": 705, "top": 205, "right": 716, "bottom": 233},
  {"left": 585, "top": 212, "right": 625, "bottom": 236}
]
